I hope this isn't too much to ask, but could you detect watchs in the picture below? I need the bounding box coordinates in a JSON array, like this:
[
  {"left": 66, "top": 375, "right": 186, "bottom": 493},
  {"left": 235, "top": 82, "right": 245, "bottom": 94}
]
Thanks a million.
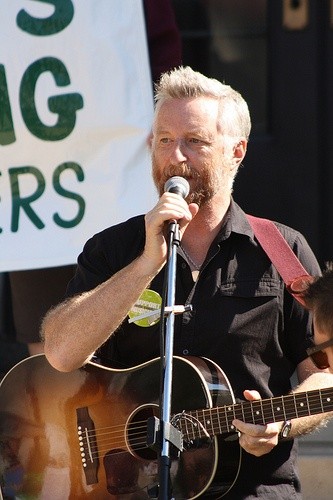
[{"left": 279, "top": 420, "right": 291, "bottom": 439}]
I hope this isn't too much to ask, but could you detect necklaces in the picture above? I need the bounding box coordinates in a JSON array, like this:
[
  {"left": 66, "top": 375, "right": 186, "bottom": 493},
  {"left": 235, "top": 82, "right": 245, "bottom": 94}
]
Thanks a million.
[{"left": 178, "top": 243, "right": 205, "bottom": 270}]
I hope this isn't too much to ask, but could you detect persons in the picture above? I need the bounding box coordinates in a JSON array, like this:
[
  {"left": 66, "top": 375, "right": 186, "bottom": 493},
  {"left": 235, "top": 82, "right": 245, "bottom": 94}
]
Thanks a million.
[
  {"left": 38, "top": 65, "right": 332, "bottom": 500},
  {"left": 297, "top": 262, "right": 333, "bottom": 374}
]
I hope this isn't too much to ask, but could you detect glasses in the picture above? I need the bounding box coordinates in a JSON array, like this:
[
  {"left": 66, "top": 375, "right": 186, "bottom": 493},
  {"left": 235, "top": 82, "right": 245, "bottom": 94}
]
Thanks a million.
[{"left": 306, "top": 339, "right": 333, "bottom": 370}]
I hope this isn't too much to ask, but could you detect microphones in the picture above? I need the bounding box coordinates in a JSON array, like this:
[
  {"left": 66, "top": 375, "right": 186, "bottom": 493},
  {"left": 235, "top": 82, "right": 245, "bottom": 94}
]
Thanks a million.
[{"left": 163, "top": 175, "right": 191, "bottom": 234}]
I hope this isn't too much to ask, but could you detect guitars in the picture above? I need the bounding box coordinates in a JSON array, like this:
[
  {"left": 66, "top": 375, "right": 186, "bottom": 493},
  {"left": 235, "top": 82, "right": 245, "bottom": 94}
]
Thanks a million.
[{"left": 0, "top": 351, "right": 333, "bottom": 500}]
